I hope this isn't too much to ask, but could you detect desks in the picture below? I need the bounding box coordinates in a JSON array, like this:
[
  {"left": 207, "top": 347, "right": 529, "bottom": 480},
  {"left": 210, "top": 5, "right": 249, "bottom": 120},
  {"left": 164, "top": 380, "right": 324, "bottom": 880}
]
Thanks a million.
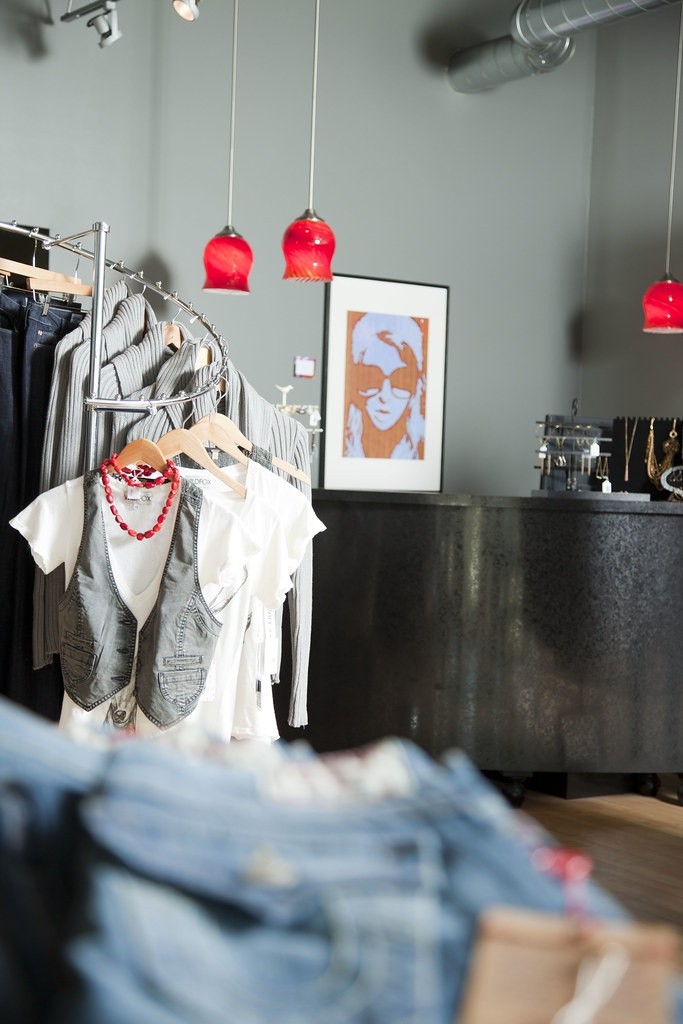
[{"left": 276, "top": 488, "right": 683, "bottom": 800}]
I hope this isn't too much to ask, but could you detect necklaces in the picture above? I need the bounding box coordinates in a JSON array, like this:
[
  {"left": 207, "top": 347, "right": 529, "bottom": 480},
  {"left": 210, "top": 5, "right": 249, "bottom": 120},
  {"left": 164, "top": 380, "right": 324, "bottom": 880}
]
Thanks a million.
[
  {"left": 644, "top": 416, "right": 681, "bottom": 488},
  {"left": 624, "top": 415, "right": 638, "bottom": 480},
  {"left": 99, "top": 445, "right": 178, "bottom": 541}
]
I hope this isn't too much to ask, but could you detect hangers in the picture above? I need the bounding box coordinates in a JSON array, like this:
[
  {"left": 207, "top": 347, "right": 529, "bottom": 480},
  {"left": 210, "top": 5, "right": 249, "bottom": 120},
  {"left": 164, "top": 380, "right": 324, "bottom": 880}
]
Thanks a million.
[
  {"left": 187, "top": 382, "right": 249, "bottom": 466},
  {"left": 106, "top": 398, "right": 180, "bottom": 484},
  {"left": 0, "top": 228, "right": 83, "bottom": 299},
  {"left": 25, "top": 247, "right": 92, "bottom": 304},
  {"left": 190, "top": 316, "right": 213, "bottom": 371},
  {"left": 192, "top": 374, "right": 312, "bottom": 486},
  {"left": 154, "top": 394, "right": 247, "bottom": 498},
  {"left": 210, "top": 336, "right": 229, "bottom": 394},
  {"left": 160, "top": 293, "right": 182, "bottom": 350}
]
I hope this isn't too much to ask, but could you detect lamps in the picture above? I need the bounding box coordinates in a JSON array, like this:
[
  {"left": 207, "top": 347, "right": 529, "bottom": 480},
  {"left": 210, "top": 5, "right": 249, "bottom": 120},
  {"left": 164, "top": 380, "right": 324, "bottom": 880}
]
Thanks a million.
[
  {"left": 282, "top": 0, "right": 335, "bottom": 283},
  {"left": 642, "top": 8, "right": 683, "bottom": 336},
  {"left": 172, "top": 0, "right": 200, "bottom": 22},
  {"left": 200, "top": 0, "right": 253, "bottom": 296},
  {"left": 60, "top": 0, "right": 123, "bottom": 50}
]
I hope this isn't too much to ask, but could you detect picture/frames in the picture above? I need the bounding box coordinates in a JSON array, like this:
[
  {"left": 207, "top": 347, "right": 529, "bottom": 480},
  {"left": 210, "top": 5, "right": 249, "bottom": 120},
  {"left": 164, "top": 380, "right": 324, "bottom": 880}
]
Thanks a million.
[{"left": 317, "top": 274, "right": 450, "bottom": 492}]
